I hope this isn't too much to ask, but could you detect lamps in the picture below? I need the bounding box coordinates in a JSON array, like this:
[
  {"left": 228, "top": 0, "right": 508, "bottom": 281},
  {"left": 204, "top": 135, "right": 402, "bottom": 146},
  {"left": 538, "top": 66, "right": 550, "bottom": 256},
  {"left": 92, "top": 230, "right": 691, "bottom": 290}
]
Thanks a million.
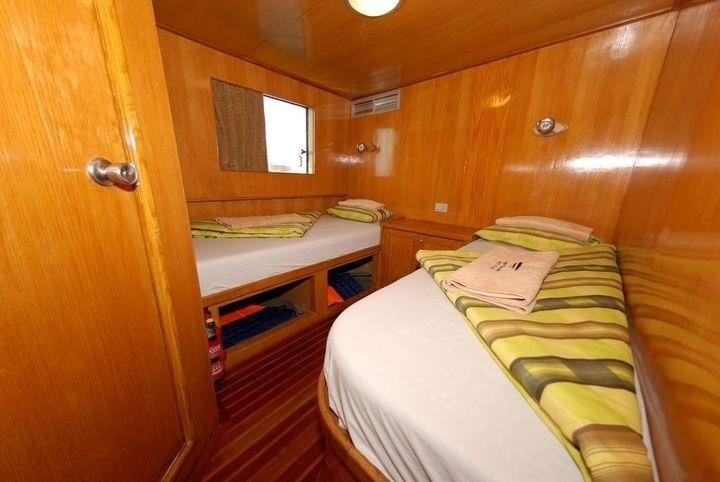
[{"left": 529, "top": 114, "right": 570, "bottom": 138}]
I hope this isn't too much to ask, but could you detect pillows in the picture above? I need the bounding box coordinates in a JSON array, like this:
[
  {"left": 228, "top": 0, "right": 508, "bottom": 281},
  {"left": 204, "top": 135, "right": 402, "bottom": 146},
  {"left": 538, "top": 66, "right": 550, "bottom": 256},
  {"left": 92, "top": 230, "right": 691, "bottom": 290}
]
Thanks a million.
[
  {"left": 327, "top": 203, "right": 394, "bottom": 224},
  {"left": 472, "top": 223, "right": 608, "bottom": 252}
]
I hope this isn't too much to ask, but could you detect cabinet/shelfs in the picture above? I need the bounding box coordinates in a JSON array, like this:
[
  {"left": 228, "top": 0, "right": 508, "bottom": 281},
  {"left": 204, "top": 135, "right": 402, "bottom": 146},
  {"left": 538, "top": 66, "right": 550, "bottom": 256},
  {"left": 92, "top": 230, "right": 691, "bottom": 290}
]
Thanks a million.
[
  {"left": 382, "top": 228, "right": 464, "bottom": 284},
  {"left": 213, "top": 251, "right": 379, "bottom": 362}
]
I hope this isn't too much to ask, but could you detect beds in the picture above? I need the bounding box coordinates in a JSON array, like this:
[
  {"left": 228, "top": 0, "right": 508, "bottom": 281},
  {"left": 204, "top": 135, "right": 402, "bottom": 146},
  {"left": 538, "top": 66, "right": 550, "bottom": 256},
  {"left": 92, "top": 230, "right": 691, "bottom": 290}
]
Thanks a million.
[
  {"left": 191, "top": 213, "right": 384, "bottom": 301},
  {"left": 316, "top": 226, "right": 664, "bottom": 481}
]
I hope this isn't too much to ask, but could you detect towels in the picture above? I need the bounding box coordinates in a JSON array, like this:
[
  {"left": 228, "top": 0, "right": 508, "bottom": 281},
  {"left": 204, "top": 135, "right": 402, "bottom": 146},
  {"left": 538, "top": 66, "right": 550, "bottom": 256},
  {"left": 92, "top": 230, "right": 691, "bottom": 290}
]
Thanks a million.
[
  {"left": 213, "top": 213, "right": 311, "bottom": 231},
  {"left": 441, "top": 247, "right": 560, "bottom": 315}
]
